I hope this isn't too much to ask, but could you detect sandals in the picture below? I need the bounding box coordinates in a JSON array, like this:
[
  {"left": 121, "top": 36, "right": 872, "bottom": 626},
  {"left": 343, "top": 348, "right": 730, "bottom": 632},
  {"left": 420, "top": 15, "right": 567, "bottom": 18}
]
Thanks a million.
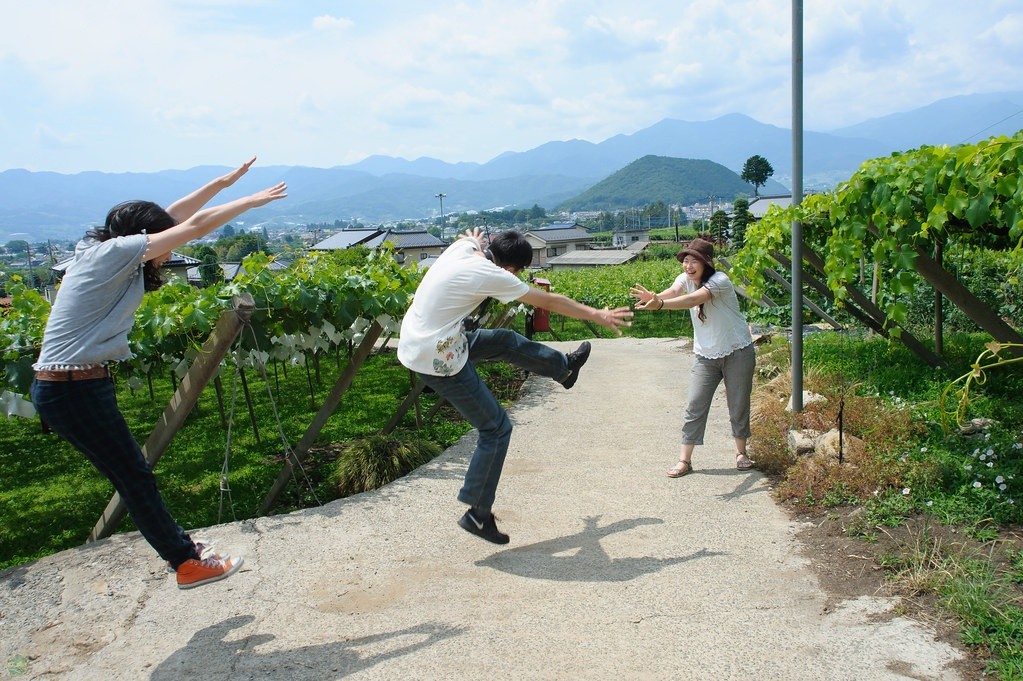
[
  {"left": 668, "top": 459, "right": 693, "bottom": 478},
  {"left": 736, "top": 451, "right": 753, "bottom": 470}
]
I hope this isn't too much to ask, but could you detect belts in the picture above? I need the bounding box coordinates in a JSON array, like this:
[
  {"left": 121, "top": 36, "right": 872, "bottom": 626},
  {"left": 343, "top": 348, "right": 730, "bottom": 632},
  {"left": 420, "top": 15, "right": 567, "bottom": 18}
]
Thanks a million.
[{"left": 36, "top": 368, "right": 110, "bottom": 382}]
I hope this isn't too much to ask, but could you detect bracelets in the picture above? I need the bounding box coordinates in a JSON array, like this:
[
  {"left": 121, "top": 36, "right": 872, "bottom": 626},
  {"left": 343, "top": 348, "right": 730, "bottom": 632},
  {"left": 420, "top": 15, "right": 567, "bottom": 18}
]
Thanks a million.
[{"left": 657, "top": 299, "right": 664, "bottom": 310}]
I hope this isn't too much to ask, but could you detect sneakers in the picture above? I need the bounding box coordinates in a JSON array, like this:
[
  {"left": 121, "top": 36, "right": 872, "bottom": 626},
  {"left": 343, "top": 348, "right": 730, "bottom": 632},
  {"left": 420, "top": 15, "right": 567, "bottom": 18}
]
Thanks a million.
[
  {"left": 561, "top": 341, "right": 591, "bottom": 389},
  {"left": 457, "top": 509, "right": 509, "bottom": 544},
  {"left": 165, "top": 538, "right": 231, "bottom": 574},
  {"left": 176, "top": 546, "right": 244, "bottom": 589}
]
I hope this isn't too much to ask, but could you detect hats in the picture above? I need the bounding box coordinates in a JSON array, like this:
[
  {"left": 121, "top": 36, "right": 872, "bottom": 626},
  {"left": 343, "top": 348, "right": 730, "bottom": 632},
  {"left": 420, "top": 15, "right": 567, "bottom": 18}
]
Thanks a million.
[{"left": 677, "top": 238, "right": 714, "bottom": 270}]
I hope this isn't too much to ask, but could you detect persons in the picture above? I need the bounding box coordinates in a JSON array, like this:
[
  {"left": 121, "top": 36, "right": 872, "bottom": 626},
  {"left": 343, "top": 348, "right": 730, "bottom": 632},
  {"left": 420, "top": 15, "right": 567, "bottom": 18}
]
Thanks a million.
[
  {"left": 396, "top": 228, "right": 633, "bottom": 544},
  {"left": 33, "top": 155, "right": 288, "bottom": 590},
  {"left": 630, "top": 239, "right": 756, "bottom": 478}
]
